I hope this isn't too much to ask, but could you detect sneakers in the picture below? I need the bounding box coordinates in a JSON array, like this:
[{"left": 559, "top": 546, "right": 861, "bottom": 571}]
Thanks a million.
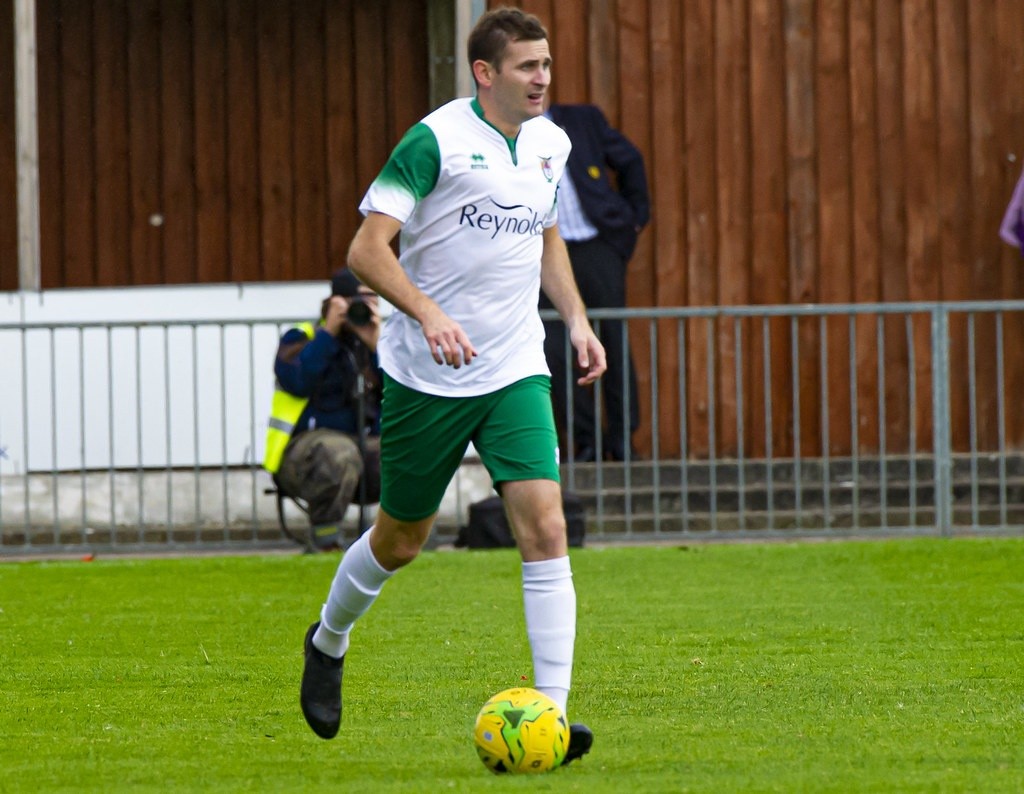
[
  {"left": 560, "top": 724, "right": 594, "bottom": 766},
  {"left": 300, "top": 621, "right": 347, "bottom": 740}
]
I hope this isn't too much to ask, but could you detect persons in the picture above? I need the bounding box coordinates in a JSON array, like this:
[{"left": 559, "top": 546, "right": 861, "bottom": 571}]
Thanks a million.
[
  {"left": 538, "top": 95, "right": 650, "bottom": 462},
  {"left": 261, "top": 268, "right": 380, "bottom": 557},
  {"left": 298, "top": 3, "right": 606, "bottom": 768}
]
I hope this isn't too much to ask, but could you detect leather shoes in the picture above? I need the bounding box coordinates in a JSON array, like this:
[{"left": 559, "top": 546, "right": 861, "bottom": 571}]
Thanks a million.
[{"left": 575, "top": 433, "right": 639, "bottom": 462}]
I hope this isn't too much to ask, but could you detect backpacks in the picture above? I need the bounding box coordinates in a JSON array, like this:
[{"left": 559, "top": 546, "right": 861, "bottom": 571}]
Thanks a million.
[{"left": 454, "top": 496, "right": 587, "bottom": 548}]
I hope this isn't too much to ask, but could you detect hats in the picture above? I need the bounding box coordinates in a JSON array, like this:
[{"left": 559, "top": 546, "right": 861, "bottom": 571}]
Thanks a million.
[{"left": 332, "top": 268, "right": 378, "bottom": 295}]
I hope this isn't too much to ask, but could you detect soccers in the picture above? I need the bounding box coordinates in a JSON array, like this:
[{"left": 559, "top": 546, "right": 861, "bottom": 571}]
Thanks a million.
[{"left": 474, "top": 688, "right": 571, "bottom": 775}]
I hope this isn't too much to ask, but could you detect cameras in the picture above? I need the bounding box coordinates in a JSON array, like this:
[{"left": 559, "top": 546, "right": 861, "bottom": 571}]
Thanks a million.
[{"left": 320, "top": 294, "right": 378, "bottom": 323}]
